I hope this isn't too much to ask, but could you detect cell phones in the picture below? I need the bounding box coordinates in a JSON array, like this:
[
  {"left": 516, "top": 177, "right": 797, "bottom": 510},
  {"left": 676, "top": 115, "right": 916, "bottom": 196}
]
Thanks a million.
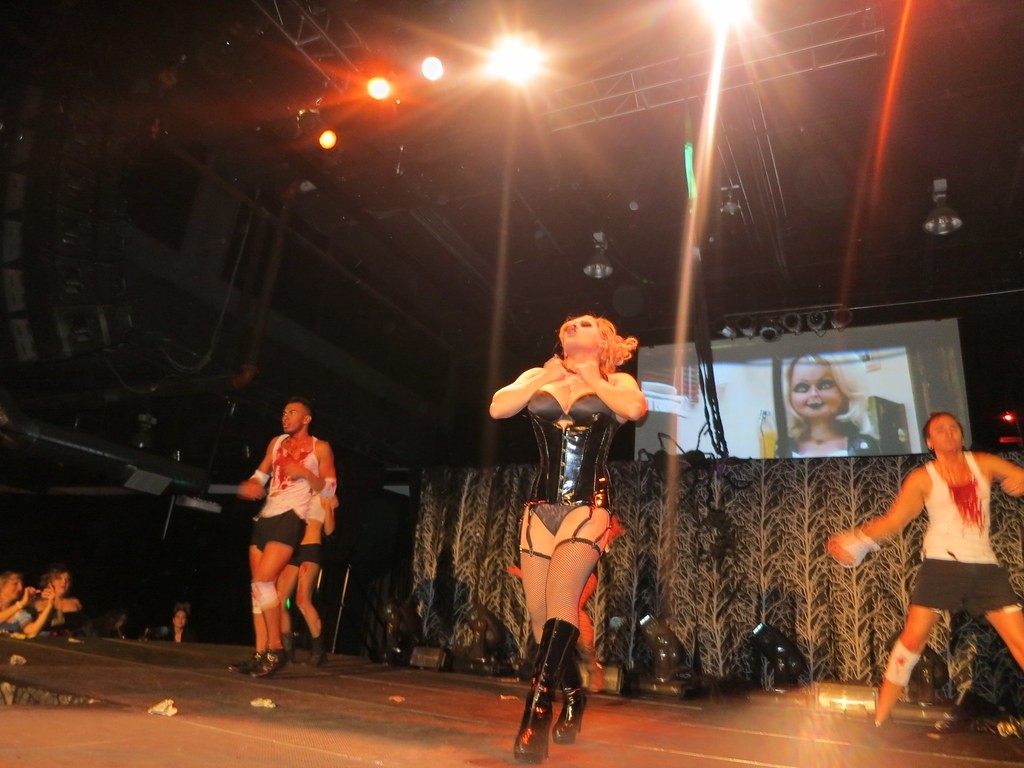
[{"left": 30, "top": 586, "right": 43, "bottom": 600}]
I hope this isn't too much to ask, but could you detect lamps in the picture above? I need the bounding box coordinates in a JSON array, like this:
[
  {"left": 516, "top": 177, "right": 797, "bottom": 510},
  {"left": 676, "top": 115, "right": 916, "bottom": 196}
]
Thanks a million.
[
  {"left": 923, "top": 176, "right": 965, "bottom": 235},
  {"left": 583, "top": 231, "right": 613, "bottom": 279},
  {"left": 720, "top": 183, "right": 742, "bottom": 220}
]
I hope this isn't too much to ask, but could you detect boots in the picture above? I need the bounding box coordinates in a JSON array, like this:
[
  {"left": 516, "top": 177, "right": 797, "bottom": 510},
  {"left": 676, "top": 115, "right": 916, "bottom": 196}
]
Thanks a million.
[
  {"left": 280, "top": 631, "right": 295, "bottom": 663},
  {"left": 539, "top": 643, "right": 587, "bottom": 744},
  {"left": 513, "top": 617, "right": 581, "bottom": 764},
  {"left": 308, "top": 633, "right": 327, "bottom": 666}
]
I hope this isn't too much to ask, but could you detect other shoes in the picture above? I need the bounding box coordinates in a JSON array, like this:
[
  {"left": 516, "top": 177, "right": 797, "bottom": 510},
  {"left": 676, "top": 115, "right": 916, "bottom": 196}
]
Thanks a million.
[{"left": 239, "top": 649, "right": 286, "bottom": 678}]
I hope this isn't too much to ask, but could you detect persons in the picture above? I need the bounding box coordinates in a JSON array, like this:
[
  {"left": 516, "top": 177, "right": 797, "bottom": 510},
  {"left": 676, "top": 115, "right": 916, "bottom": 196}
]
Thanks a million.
[
  {"left": 227, "top": 398, "right": 339, "bottom": 677},
  {"left": 490, "top": 313, "right": 647, "bottom": 759},
  {"left": 163, "top": 606, "right": 192, "bottom": 643},
  {"left": 0, "top": 566, "right": 127, "bottom": 640},
  {"left": 775, "top": 352, "right": 880, "bottom": 457},
  {"left": 830, "top": 412, "right": 1024, "bottom": 743}
]
